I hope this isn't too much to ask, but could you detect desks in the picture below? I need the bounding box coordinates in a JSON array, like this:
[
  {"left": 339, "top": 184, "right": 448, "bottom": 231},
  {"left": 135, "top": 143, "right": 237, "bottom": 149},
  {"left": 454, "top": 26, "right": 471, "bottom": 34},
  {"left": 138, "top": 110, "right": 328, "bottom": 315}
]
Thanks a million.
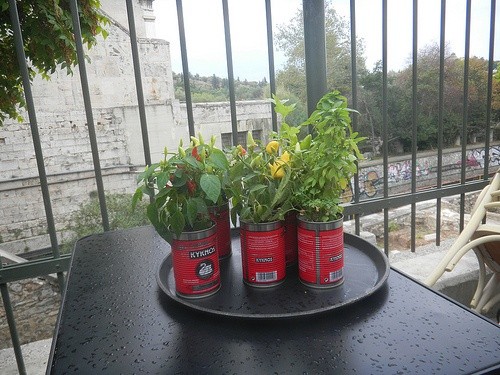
[{"left": 46, "top": 227, "right": 500, "bottom": 374}]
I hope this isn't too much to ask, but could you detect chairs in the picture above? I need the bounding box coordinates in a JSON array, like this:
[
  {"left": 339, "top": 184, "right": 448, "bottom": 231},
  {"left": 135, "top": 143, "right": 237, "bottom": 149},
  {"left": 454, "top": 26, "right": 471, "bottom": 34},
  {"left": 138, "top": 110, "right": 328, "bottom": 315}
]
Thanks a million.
[{"left": 427, "top": 167, "right": 500, "bottom": 318}]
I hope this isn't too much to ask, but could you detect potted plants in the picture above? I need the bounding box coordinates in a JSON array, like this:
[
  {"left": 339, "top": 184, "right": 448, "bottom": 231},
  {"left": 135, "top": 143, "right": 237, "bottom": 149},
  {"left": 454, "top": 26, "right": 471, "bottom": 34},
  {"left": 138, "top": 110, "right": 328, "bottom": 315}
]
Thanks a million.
[{"left": 129, "top": 90, "right": 369, "bottom": 300}]
